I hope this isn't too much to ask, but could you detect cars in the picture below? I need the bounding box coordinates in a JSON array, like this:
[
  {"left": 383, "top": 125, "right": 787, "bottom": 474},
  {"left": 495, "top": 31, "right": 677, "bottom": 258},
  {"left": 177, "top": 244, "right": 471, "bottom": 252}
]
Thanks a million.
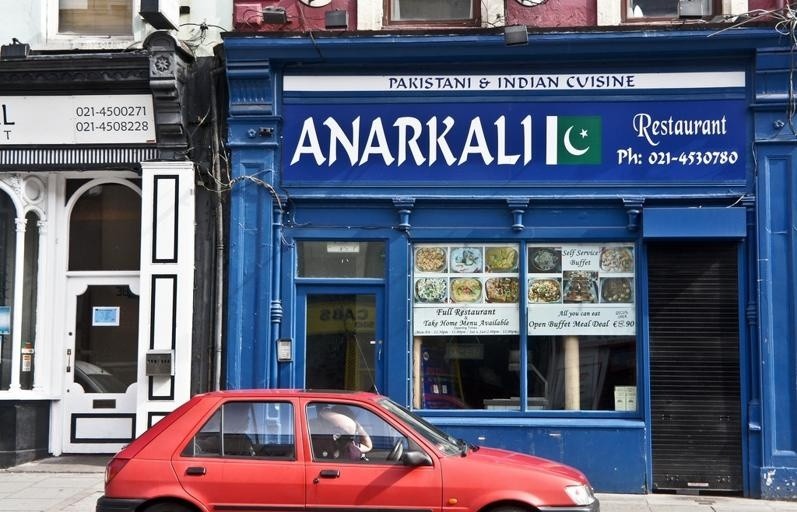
[{"left": 96, "top": 389, "right": 599, "bottom": 512}]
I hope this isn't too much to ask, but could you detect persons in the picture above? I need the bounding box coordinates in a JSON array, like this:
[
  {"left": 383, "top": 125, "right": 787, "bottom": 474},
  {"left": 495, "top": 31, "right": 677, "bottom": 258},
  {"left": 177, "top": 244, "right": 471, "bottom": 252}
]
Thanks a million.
[
  {"left": 463, "top": 338, "right": 520, "bottom": 409},
  {"left": 217, "top": 403, "right": 257, "bottom": 457},
  {"left": 596, "top": 342, "right": 636, "bottom": 410},
  {"left": 315, "top": 404, "right": 373, "bottom": 462}
]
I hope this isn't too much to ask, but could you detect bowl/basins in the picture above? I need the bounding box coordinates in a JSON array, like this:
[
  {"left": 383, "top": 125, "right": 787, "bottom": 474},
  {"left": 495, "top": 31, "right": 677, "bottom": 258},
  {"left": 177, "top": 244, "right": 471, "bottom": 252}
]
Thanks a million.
[{"left": 531, "top": 250, "right": 560, "bottom": 272}]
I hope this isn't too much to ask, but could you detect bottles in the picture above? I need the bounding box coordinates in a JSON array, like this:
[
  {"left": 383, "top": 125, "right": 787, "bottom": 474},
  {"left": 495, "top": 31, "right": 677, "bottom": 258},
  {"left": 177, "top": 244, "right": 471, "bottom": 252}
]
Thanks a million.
[{"left": 20, "top": 341, "right": 34, "bottom": 390}]
